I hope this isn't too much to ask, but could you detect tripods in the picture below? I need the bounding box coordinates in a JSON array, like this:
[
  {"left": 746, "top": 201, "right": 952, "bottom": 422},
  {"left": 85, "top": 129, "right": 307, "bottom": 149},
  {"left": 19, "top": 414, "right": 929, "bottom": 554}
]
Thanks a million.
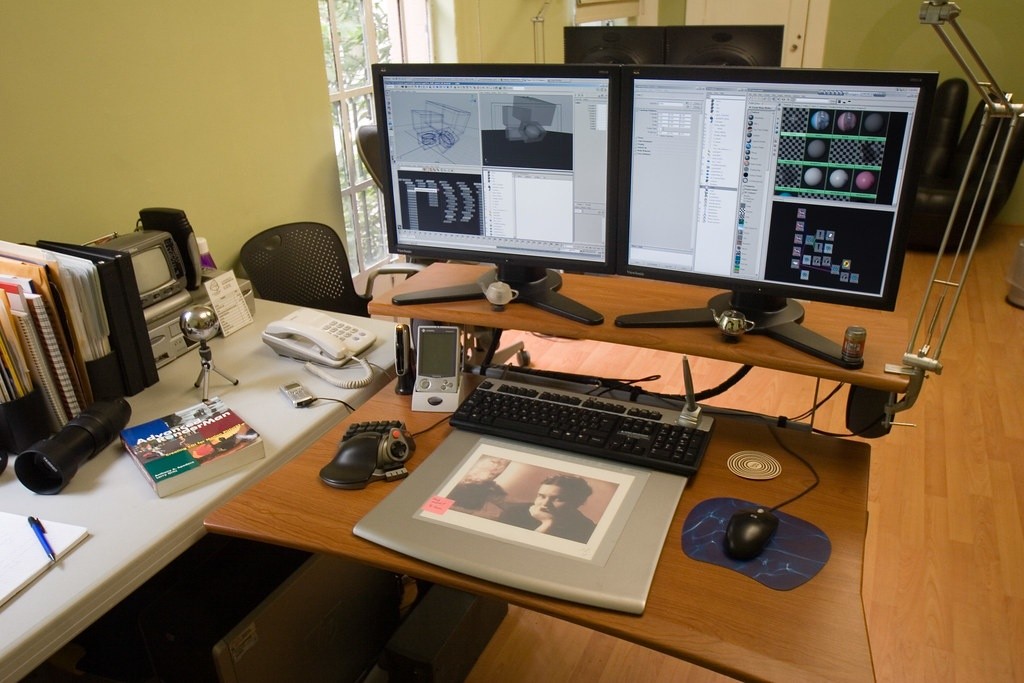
[{"left": 194, "top": 340, "right": 239, "bottom": 402}]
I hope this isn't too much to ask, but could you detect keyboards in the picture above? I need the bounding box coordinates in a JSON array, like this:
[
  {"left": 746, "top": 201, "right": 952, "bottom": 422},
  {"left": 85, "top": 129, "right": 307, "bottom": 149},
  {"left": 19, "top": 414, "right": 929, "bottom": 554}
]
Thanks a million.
[{"left": 449, "top": 378, "right": 715, "bottom": 478}]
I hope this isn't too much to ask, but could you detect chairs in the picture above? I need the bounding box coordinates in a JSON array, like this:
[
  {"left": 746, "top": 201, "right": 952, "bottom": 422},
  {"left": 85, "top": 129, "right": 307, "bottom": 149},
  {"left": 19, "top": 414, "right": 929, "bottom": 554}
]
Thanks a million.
[
  {"left": 239, "top": 222, "right": 426, "bottom": 318},
  {"left": 906, "top": 79, "right": 1024, "bottom": 251}
]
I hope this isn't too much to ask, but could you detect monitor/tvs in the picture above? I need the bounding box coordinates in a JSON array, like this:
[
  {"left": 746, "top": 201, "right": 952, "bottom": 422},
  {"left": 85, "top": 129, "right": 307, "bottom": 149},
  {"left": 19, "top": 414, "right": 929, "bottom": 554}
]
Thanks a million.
[
  {"left": 372, "top": 63, "right": 622, "bottom": 325},
  {"left": 616, "top": 64, "right": 940, "bottom": 370},
  {"left": 562, "top": 25, "right": 784, "bottom": 67},
  {"left": 102, "top": 232, "right": 188, "bottom": 309}
]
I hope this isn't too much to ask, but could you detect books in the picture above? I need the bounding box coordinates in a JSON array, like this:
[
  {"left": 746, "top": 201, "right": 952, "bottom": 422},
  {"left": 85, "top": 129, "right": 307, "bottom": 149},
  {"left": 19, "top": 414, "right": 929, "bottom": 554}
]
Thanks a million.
[
  {"left": 0, "top": 511, "right": 89, "bottom": 606},
  {"left": 0, "top": 241, "right": 112, "bottom": 431},
  {"left": 120, "top": 396, "right": 264, "bottom": 497}
]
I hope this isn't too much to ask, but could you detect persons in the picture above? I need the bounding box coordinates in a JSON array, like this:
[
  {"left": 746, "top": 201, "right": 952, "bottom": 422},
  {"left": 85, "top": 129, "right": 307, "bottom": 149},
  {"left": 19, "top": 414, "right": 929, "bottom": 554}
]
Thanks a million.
[
  {"left": 444, "top": 455, "right": 507, "bottom": 521},
  {"left": 499, "top": 453, "right": 595, "bottom": 545}
]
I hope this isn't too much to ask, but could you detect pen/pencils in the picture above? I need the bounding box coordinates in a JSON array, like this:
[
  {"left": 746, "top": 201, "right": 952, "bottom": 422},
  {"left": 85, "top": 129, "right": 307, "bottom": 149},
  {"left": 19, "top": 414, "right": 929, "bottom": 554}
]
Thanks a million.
[{"left": 26, "top": 515, "right": 58, "bottom": 567}]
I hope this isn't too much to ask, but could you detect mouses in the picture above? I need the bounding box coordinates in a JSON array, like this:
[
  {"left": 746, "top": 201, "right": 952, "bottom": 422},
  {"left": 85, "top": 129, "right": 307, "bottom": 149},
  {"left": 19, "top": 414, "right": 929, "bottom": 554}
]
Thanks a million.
[{"left": 723, "top": 508, "right": 779, "bottom": 561}]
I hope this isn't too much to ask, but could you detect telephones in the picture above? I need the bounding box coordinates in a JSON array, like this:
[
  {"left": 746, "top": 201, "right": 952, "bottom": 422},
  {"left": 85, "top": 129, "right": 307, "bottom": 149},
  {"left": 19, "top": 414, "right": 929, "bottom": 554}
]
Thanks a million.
[{"left": 262, "top": 306, "right": 376, "bottom": 370}]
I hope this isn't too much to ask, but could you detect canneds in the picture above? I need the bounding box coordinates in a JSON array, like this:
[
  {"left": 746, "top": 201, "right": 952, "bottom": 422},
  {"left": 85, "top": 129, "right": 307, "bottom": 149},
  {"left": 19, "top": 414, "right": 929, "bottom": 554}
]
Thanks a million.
[{"left": 842, "top": 325, "right": 867, "bottom": 361}]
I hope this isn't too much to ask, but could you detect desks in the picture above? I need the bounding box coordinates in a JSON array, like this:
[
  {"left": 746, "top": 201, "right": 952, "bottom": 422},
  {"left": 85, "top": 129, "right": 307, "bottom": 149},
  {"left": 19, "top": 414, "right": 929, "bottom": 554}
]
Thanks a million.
[
  {"left": 0, "top": 298, "right": 403, "bottom": 683},
  {"left": 203, "top": 262, "right": 876, "bottom": 682}
]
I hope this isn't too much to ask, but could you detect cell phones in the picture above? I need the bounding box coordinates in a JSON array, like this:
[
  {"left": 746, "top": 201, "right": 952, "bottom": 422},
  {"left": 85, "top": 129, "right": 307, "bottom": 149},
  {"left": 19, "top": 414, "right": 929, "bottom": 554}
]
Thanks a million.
[{"left": 279, "top": 380, "right": 313, "bottom": 407}]
